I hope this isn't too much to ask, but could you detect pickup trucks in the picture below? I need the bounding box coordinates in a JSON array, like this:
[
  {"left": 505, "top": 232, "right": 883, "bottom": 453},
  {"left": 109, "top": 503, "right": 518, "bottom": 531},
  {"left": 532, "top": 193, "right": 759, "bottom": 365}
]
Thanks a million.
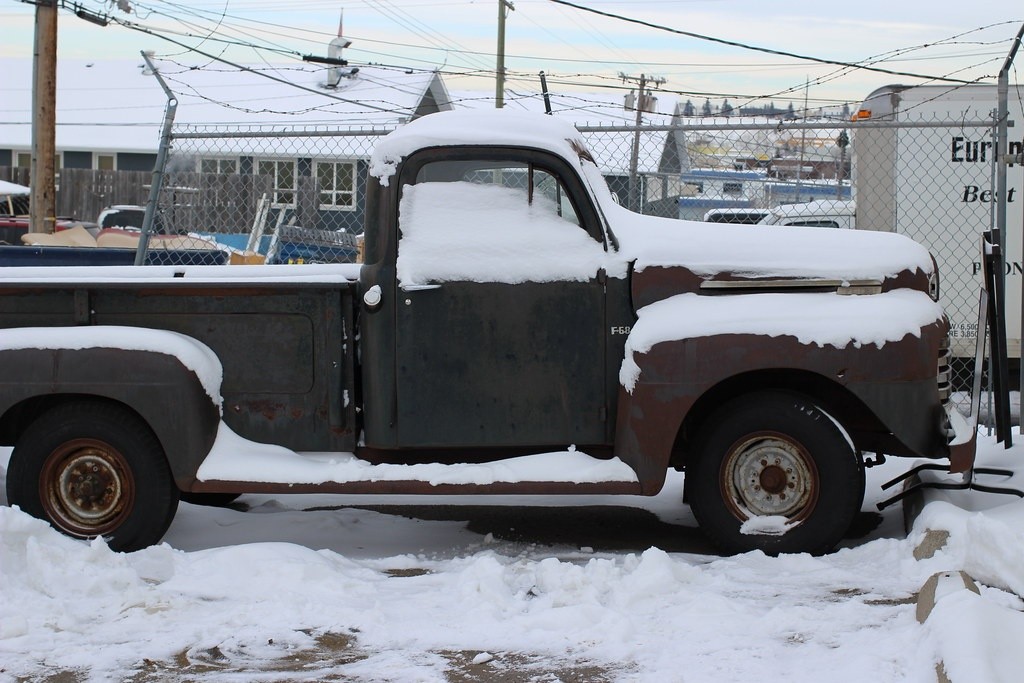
[{"left": 0, "top": 110, "right": 975, "bottom": 552}]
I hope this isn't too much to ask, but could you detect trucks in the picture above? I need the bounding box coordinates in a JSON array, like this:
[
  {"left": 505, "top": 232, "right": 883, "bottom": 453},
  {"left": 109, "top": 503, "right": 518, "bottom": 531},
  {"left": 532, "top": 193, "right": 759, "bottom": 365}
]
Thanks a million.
[{"left": 753, "top": 84, "right": 1024, "bottom": 359}]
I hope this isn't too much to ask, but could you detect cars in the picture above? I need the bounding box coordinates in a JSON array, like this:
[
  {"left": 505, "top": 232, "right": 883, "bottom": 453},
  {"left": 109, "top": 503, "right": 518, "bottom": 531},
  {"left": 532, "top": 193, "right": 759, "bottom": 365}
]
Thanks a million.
[
  {"left": 96, "top": 203, "right": 189, "bottom": 236},
  {"left": 0, "top": 215, "right": 101, "bottom": 246}
]
